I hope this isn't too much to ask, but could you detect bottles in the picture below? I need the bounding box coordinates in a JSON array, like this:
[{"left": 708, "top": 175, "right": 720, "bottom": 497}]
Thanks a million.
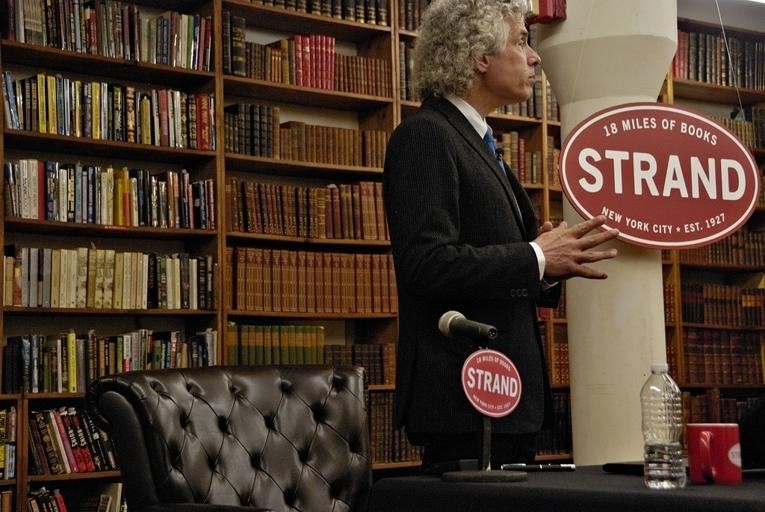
[{"left": 638, "top": 363, "right": 689, "bottom": 491}]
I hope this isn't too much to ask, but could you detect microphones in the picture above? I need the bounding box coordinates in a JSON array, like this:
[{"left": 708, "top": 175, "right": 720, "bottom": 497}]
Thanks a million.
[{"left": 436, "top": 308, "right": 498, "bottom": 340}]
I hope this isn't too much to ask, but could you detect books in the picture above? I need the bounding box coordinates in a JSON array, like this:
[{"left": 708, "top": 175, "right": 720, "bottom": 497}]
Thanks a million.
[
  {"left": 212, "top": 0, "right": 420, "bottom": 465},
  {"left": 656, "top": 20, "right": 765, "bottom": 481},
  {"left": 395, "top": 1, "right": 571, "bottom": 460},
  {"left": 3, "top": 2, "right": 214, "bottom": 509}
]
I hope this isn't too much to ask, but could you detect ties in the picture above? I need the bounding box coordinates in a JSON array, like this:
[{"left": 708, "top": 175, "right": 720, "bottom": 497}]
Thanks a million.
[{"left": 485, "top": 127, "right": 506, "bottom": 176}]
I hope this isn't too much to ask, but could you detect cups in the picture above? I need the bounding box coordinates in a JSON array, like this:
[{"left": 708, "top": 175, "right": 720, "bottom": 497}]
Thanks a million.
[{"left": 682, "top": 421, "right": 743, "bottom": 487}]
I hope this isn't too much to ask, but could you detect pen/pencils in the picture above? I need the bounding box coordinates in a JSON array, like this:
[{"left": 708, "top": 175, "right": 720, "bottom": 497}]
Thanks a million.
[{"left": 500, "top": 463, "right": 575, "bottom": 471}]
